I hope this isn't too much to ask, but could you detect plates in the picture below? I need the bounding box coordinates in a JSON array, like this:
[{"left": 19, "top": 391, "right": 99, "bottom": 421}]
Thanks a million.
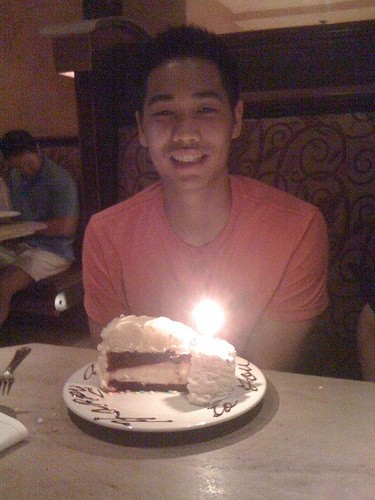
[
  {"left": 62, "top": 356, "right": 267, "bottom": 431},
  {"left": 0, "top": 211, "right": 22, "bottom": 221}
]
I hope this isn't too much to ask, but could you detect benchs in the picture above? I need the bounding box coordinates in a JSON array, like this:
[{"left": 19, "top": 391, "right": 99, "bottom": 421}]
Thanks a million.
[
  {"left": 11, "top": 135, "right": 83, "bottom": 320},
  {"left": 118, "top": 86, "right": 375, "bottom": 382}
]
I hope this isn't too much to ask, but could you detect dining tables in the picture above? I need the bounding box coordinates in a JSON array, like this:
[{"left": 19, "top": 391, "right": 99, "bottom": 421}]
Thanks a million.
[{"left": 0, "top": 341, "right": 375, "bottom": 500}]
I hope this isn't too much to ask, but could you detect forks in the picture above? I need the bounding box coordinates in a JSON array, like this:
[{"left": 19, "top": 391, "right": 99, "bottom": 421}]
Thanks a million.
[{"left": 0, "top": 347, "right": 31, "bottom": 395}]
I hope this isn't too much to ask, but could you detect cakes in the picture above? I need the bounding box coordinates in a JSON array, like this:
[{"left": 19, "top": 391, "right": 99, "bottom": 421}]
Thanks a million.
[{"left": 93, "top": 314, "right": 237, "bottom": 406}]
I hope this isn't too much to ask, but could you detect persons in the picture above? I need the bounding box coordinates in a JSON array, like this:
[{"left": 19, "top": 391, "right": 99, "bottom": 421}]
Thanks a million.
[
  {"left": 83, "top": 26, "right": 333, "bottom": 371},
  {"left": 356, "top": 230, "right": 375, "bottom": 383},
  {"left": 0, "top": 130, "right": 78, "bottom": 328}
]
